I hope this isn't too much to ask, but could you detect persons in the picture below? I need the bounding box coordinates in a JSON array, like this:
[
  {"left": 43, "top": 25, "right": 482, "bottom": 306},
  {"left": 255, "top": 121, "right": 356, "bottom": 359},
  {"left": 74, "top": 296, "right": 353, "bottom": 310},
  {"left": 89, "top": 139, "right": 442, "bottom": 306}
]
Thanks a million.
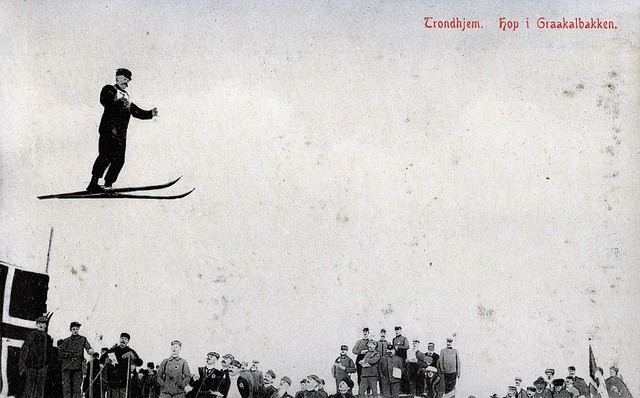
[
  {"left": 357, "top": 340, "right": 380, "bottom": 398},
  {"left": 393, "top": 327, "right": 409, "bottom": 359},
  {"left": 83, "top": 332, "right": 329, "bottom": 398},
  {"left": 490, "top": 366, "right": 633, "bottom": 398},
  {"left": 405, "top": 339, "right": 433, "bottom": 398},
  {"left": 352, "top": 328, "right": 373, "bottom": 397},
  {"left": 59, "top": 322, "right": 98, "bottom": 397},
  {"left": 378, "top": 345, "right": 404, "bottom": 398},
  {"left": 376, "top": 329, "right": 391, "bottom": 395},
  {"left": 418, "top": 366, "right": 442, "bottom": 398},
  {"left": 331, "top": 346, "right": 357, "bottom": 395},
  {"left": 328, "top": 376, "right": 355, "bottom": 398},
  {"left": 439, "top": 338, "right": 461, "bottom": 398},
  {"left": 87, "top": 68, "right": 160, "bottom": 192},
  {"left": 17, "top": 316, "right": 54, "bottom": 397},
  {"left": 424, "top": 342, "right": 439, "bottom": 368}
]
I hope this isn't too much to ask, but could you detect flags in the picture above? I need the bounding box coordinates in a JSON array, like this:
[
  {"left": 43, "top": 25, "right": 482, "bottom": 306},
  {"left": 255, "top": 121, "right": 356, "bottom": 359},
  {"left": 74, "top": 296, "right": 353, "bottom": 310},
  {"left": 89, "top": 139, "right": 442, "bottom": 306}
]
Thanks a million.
[{"left": 588, "top": 337, "right": 596, "bottom": 378}]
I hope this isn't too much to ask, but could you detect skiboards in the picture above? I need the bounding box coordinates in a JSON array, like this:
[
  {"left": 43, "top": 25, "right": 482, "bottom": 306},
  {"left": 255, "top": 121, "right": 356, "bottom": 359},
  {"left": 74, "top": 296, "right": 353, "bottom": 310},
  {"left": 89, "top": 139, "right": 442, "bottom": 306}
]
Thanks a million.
[{"left": 37, "top": 176, "right": 193, "bottom": 198}]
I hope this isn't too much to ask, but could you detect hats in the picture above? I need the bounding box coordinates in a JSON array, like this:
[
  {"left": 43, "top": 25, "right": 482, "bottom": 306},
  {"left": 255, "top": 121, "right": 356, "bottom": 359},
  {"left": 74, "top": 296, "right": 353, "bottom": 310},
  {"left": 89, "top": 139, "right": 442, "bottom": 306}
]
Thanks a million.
[
  {"left": 609, "top": 366, "right": 618, "bottom": 375},
  {"left": 386, "top": 345, "right": 394, "bottom": 350},
  {"left": 380, "top": 329, "right": 386, "bottom": 332},
  {"left": 413, "top": 339, "right": 419, "bottom": 342},
  {"left": 553, "top": 379, "right": 564, "bottom": 386},
  {"left": 568, "top": 366, "right": 575, "bottom": 371},
  {"left": 527, "top": 387, "right": 536, "bottom": 392},
  {"left": 120, "top": 333, "right": 130, "bottom": 341},
  {"left": 367, "top": 339, "right": 378, "bottom": 346},
  {"left": 341, "top": 377, "right": 354, "bottom": 390},
  {"left": 207, "top": 352, "right": 219, "bottom": 360},
  {"left": 509, "top": 386, "right": 517, "bottom": 390},
  {"left": 533, "top": 377, "right": 547, "bottom": 385},
  {"left": 395, "top": 327, "right": 402, "bottom": 330},
  {"left": 230, "top": 360, "right": 241, "bottom": 368},
  {"left": 116, "top": 68, "right": 132, "bottom": 81},
  {"left": 341, "top": 345, "right": 348, "bottom": 351},
  {"left": 545, "top": 369, "right": 554, "bottom": 375},
  {"left": 101, "top": 348, "right": 109, "bottom": 352},
  {"left": 446, "top": 338, "right": 453, "bottom": 342},
  {"left": 171, "top": 340, "right": 181, "bottom": 346},
  {"left": 70, "top": 322, "right": 81, "bottom": 331},
  {"left": 300, "top": 379, "right": 307, "bottom": 384},
  {"left": 241, "top": 361, "right": 248, "bottom": 365},
  {"left": 266, "top": 370, "right": 275, "bottom": 378},
  {"left": 363, "top": 328, "right": 369, "bottom": 332},
  {"left": 307, "top": 375, "right": 320, "bottom": 384},
  {"left": 35, "top": 317, "right": 48, "bottom": 322},
  {"left": 252, "top": 360, "right": 259, "bottom": 364},
  {"left": 222, "top": 354, "right": 234, "bottom": 361},
  {"left": 566, "top": 376, "right": 574, "bottom": 381},
  {"left": 92, "top": 353, "right": 99, "bottom": 358},
  {"left": 428, "top": 342, "right": 434, "bottom": 346},
  {"left": 280, "top": 376, "right": 291, "bottom": 385},
  {"left": 147, "top": 362, "right": 154, "bottom": 369},
  {"left": 515, "top": 377, "right": 522, "bottom": 382},
  {"left": 426, "top": 366, "right": 437, "bottom": 373}
]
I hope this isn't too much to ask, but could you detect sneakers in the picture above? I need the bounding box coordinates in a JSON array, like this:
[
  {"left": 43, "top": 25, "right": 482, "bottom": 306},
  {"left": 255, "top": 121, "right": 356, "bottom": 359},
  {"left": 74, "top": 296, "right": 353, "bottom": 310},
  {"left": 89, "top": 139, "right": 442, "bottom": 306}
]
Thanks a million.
[
  {"left": 104, "top": 186, "right": 118, "bottom": 196},
  {"left": 86, "top": 176, "right": 104, "bottom": 191}
]
